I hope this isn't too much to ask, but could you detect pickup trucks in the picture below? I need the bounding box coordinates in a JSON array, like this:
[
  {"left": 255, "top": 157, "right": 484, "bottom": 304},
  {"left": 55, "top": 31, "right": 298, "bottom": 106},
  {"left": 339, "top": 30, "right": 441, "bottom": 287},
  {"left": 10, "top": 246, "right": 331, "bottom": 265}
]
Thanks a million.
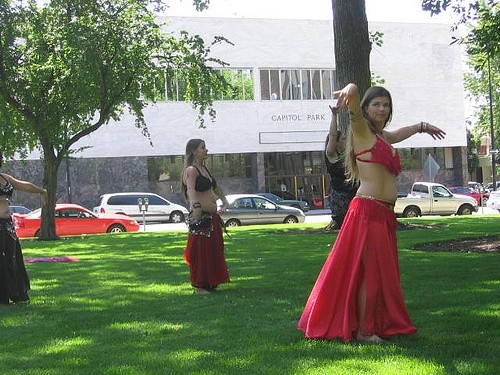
[{"left": 394, "top": 181, "right": 479, "bottom": 219}]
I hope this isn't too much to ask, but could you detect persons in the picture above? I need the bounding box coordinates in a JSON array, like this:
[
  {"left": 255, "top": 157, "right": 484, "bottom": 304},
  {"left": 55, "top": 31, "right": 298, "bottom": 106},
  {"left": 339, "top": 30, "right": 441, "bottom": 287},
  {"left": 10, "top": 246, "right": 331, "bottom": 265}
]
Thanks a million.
[
  {"left": 324, "top": 105, "right": 361, "bottom": 229},
  {"left": 0, "top": 152, "right": 47, "bottom": 303},
  {"left": 296, "top": 82, "right": 446, "bottom": 343},
  {"left": 183, "top": 139, "right": 230, "bottom": 295}
]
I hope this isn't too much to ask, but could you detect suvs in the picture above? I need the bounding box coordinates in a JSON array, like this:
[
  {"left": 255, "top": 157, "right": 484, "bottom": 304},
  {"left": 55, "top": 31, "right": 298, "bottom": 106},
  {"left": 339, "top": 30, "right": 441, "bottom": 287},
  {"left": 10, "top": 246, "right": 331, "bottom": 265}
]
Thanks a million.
[
  {"left": 256, "top": 192, "right": 310, "bottom": 213},
  {"left": 468, "top": 182, "right": 490, "bottom": 200}
]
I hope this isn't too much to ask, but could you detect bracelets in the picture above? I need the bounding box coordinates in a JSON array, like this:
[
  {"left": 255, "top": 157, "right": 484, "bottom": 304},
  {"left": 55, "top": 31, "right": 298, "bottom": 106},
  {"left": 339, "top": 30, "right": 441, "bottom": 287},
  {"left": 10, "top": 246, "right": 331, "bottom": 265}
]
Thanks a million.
[
  {"left": 418, "top": 122, "right": 427, "bottom": 133},
  {"left": 193, "top": 202, "right": 201, "bottom": 208}
]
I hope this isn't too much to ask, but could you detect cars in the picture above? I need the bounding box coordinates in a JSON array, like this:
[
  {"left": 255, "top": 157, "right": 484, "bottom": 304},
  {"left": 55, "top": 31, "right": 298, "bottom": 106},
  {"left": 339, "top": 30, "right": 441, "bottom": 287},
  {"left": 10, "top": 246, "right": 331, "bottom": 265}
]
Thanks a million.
[
  {"left": 486, "top": 181, "right": 500, "bottom": 190},
  {"left": 293, "top": 191, "right": 329, "bottom": 207},
  {"left": 448, "top": 186, "right": 490, "bottom": 207},
  {"left": 216, "top": 194, "right": 305, "bottom": 227},
  {"left": 12, "top": 203, "right": 140, "bottom": 238},
  {"left": 9, "top": 206, "right": 31, "bottom": 215}
]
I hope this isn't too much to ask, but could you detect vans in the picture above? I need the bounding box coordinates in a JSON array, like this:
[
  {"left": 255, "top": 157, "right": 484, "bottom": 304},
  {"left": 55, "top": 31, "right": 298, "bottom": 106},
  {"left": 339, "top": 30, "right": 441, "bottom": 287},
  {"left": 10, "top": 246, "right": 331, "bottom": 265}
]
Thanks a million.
[{"left": 93, "top": 192, "right": 189, "bottom": 223}]
